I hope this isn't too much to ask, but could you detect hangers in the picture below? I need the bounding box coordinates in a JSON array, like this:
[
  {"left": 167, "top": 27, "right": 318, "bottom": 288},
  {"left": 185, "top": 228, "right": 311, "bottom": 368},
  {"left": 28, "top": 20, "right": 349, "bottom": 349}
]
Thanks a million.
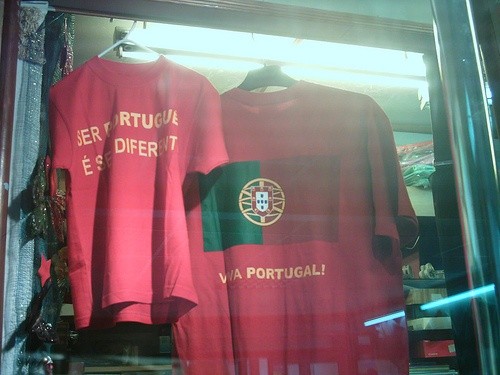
[
  {"left": 94, "top": 16, "right": 165, "bottom": 69},
  {"left": 234, "top": 34, "right": 304, "bottom": 98}
]
